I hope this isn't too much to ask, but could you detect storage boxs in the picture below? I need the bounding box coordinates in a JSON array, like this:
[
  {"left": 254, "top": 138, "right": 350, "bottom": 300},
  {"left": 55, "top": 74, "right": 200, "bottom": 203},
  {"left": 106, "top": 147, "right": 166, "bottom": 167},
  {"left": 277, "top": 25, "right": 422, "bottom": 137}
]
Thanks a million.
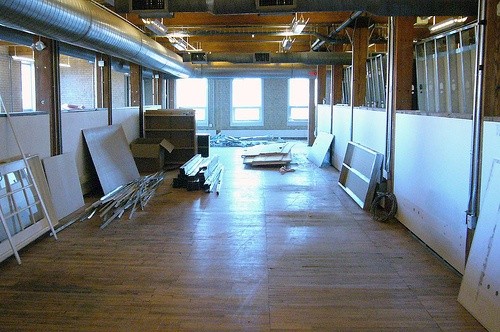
[{"left": 129, "top": 137, "right": 175, "bottom": 173}]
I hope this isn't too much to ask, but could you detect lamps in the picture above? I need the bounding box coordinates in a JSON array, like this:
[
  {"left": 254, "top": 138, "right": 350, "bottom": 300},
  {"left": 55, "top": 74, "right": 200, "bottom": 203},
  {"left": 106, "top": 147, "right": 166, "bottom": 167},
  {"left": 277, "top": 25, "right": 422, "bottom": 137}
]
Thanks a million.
[
  {"left": 142, "top": 18, "right": 168, "bottom": 36},
  {"left": 292, "top": 12, "right": 310, "bottom": 33},
  {"left": 169, "top": 25, "right": 186, "bottom": 51},
  {"left": 283, "top": 36, "right": 296, "bottom": 51},
  {"left": 33, "top": 36, "right": 46, "bottom": 50},
  {"left": 428, "top": 16, "right": 468, "bottom": 33}
]
